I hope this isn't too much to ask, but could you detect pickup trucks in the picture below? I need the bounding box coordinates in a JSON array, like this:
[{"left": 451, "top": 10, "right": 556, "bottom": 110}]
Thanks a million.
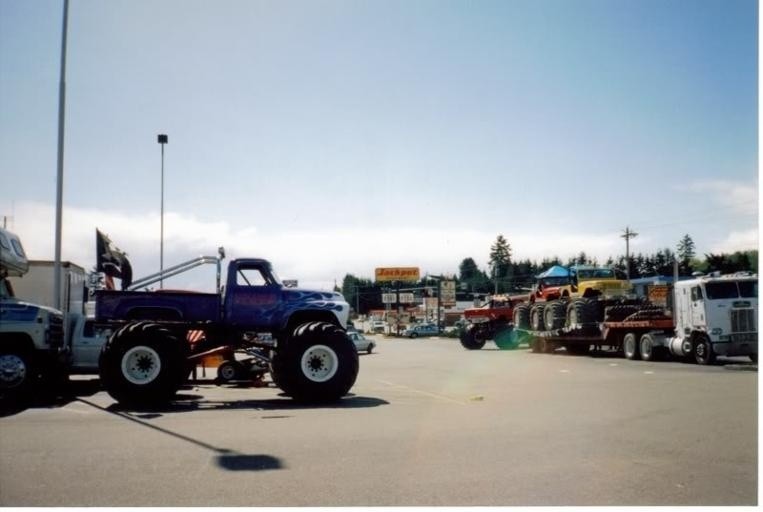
[{"left": 93, "top": 247, "right": 358, "bottom": 404}]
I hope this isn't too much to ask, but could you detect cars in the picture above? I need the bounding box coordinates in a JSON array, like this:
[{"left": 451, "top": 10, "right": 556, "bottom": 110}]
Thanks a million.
[
  {"left": 346, "top": 331, "right": 376, "bottom": 354},
  {"left": 401, "top": 323, "right": 440, "bottom": 338}
]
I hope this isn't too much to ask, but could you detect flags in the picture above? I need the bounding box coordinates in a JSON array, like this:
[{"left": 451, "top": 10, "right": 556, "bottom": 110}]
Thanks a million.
[{"left": 95, "top": 226, "right": 131, "bottom": 290}]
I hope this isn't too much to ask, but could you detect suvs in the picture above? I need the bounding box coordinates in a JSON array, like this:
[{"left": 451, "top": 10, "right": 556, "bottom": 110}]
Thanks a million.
[{"left": 460, "top": 295, "right": 530, "bottom": 349}]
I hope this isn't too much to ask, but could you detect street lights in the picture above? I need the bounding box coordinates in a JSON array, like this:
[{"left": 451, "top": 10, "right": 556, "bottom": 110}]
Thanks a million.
[{"left": 158, "top": 134, "right": 169, "bottom": 290}]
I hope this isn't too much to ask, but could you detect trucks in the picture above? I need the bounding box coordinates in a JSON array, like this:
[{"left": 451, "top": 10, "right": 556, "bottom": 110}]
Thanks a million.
[
  {"left": 0, "top": 226, "right": 64, "bottom": 393},
  {"left": 511, "top": 270, "right": 758, "bottom": 365},
  {"left": 5, "top": 261, "right": 115, "bottom": 375}
]
